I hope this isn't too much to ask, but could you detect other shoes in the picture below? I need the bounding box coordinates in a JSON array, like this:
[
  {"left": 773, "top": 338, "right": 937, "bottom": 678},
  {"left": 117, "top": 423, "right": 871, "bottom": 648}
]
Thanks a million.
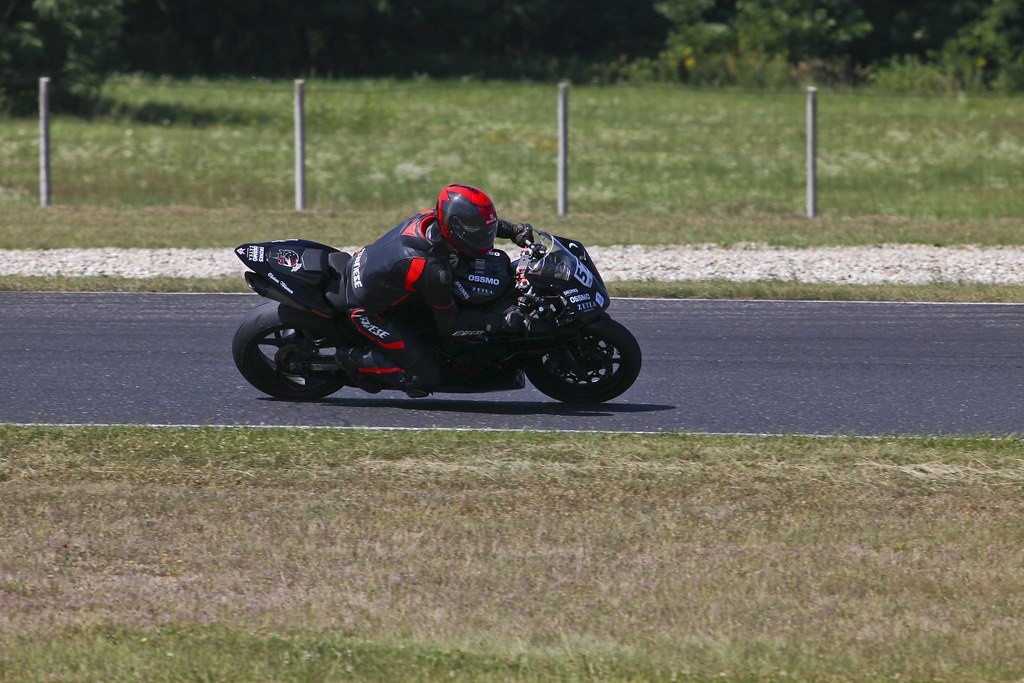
[{"left": 336, "top": 348, "right": 386, "bottom": 393}]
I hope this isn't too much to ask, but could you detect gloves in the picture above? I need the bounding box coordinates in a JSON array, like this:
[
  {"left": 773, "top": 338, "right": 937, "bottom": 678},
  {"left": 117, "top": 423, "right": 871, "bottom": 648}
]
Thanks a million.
[
  {"left": 484, "top": 311, "right": 524, "bottom": 336},
  {"left": 500, "top": 221, "right": 534, "bottom": 248}
]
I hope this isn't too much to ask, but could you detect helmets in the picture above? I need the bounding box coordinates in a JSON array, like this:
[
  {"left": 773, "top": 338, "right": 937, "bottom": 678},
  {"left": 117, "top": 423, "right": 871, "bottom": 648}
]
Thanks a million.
[{"left": 436, "top": 184, "right": 498, "bottom": 257}]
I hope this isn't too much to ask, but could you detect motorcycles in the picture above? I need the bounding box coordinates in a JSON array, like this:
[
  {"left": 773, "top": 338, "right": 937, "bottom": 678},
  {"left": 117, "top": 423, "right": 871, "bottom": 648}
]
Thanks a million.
[{"left": 234, "top": 228, "right": 643, "bottom": 411}]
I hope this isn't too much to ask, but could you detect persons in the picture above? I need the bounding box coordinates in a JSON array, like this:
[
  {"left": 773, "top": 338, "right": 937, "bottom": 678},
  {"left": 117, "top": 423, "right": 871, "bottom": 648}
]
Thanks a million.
[{"left": 345, "top": 184, "right": 534, "bottom": 398}]
